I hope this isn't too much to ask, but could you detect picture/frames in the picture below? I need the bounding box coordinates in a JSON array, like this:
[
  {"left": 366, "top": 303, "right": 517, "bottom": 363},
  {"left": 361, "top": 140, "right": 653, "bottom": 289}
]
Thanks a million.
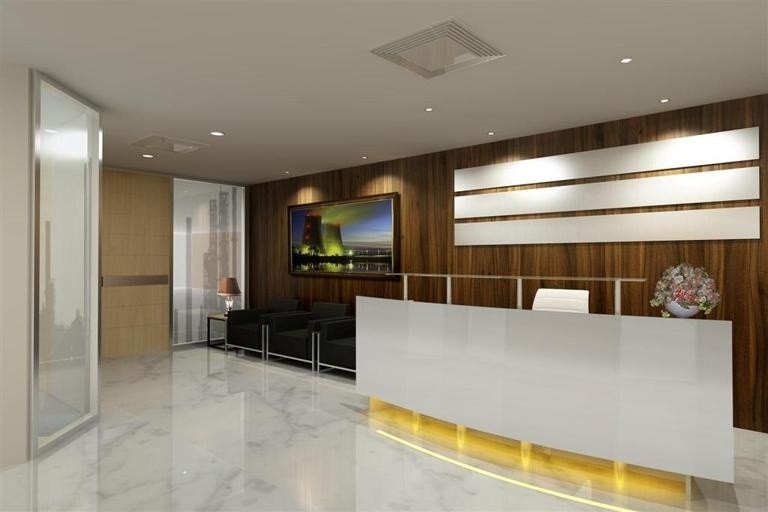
[{"left": 287, "top": 192, "right": 401, "bottom": 283}]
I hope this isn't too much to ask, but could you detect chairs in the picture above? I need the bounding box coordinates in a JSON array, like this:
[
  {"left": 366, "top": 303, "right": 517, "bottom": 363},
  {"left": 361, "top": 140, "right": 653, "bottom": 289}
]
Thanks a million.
[
  {"left": 224, "top": 299, "right": 356, "bottom": 374},
  {"left": 532, "top": 287, "right": 591, "bottom": 313}
]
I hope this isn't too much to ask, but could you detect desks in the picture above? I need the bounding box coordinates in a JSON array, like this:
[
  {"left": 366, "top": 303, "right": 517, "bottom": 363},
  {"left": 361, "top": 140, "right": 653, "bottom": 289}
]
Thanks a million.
[{"left": 207, "top": 314, "right": 239, "bottom": 351}]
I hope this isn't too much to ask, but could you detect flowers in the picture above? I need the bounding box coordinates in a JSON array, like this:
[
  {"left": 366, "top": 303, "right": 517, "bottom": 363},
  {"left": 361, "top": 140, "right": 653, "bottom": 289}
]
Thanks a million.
[{"left": 649, "top": 263, "right": 721, "bottom": 318}]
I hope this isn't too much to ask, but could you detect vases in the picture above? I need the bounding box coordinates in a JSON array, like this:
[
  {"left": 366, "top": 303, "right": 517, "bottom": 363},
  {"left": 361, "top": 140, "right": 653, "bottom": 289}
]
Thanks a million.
[{"left": 663, "top": 301, "right": 699, "bottom": 318}]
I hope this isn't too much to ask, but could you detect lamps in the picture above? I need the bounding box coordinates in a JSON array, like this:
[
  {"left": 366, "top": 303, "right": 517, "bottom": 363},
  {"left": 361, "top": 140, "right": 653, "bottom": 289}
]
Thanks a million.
[{"left": 217, "top": 278, "right": 241, "bottom": 316}]
